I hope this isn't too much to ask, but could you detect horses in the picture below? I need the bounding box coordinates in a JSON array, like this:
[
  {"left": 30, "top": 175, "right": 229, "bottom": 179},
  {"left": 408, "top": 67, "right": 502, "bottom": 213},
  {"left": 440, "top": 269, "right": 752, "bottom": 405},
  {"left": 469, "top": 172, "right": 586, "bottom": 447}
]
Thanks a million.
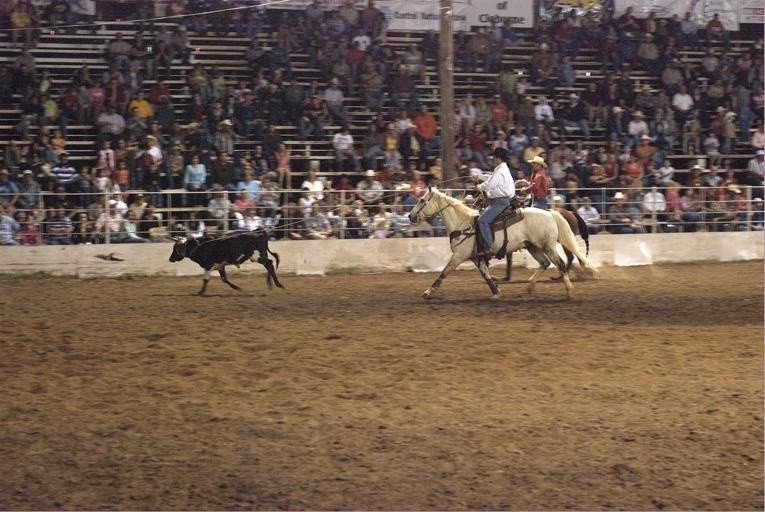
[
  {"left": 408, "top": 183, "right": 598, "bottom": 301},
  {"left": 473, "top": 191, "right": 589, "bottom": 282}
]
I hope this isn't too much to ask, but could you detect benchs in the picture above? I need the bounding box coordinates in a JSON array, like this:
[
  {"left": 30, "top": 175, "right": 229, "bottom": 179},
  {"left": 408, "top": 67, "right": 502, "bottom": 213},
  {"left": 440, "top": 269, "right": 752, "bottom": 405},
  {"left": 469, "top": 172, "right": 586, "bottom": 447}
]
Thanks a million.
[{"left": 0, "top": 0, "right": 764, "bottom": 247}]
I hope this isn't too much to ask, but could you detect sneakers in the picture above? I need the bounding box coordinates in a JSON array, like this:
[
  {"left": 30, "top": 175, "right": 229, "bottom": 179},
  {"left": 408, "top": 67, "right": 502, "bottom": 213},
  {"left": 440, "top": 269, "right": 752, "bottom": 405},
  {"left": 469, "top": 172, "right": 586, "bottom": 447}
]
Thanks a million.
[{"left": 476, "top": 242, "right": 500, "bottom": 257}]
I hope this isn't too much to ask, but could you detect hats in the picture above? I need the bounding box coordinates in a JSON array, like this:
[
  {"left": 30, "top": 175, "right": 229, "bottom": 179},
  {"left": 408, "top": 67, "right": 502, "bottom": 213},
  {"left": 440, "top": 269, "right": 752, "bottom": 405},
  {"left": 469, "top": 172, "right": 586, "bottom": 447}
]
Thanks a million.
[{"left": 0, "top": 0, "right": 765, "bottom": 217}]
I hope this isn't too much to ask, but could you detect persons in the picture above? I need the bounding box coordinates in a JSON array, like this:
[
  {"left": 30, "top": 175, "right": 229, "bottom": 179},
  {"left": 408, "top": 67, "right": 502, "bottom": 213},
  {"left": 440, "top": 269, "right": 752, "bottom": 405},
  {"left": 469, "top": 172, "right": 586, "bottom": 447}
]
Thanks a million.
[{"left": 476, "top": 147, "right": 516, "bottom": 257}]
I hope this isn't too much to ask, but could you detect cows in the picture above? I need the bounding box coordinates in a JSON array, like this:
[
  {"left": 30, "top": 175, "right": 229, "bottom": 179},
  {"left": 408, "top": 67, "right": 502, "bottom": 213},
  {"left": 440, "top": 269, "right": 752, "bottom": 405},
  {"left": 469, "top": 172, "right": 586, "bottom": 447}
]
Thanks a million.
[{"left": 168, "top": 228, "right": 285, "bottom": 295}]
